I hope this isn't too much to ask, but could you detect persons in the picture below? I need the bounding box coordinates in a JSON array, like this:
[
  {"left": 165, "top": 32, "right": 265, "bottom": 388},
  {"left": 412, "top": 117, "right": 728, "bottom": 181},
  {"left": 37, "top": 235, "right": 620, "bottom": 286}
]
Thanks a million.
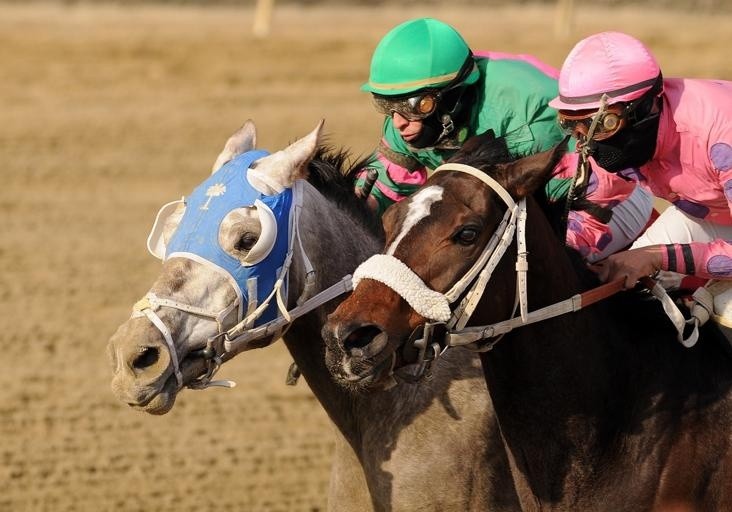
[
  {"left": 349, "top": 17, "right": 585, "bottom": 217},
  {"left": 547, "top": 30, "right": 731, "bottom": 341}
]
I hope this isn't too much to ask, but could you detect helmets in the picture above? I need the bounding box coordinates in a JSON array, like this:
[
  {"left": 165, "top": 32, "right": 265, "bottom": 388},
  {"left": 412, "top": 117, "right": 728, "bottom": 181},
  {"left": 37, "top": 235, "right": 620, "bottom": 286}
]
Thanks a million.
[
  {"left": 547, "top": 32, "right": 666, "bottom": 112},
  {"left": 361, "top": 17, "right": 482, "bottom": 97}
]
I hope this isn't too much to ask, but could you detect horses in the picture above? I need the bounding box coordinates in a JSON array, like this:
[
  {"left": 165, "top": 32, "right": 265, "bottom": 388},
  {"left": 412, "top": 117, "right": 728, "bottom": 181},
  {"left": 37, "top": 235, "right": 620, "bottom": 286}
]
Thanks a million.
[
  {"left": 103, "top": 117, "right": 524, "bottom": 512},
  {"left": 321, "top": 128, "right": 732, "bottom": 512}
]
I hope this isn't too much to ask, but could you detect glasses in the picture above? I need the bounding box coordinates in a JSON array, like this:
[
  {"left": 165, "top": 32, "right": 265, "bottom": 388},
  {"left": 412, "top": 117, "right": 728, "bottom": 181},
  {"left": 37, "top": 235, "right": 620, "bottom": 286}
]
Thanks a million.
[
  {"left": 370, "top": 50, "right": 474, "bottom": 120},
  {"left": 558, "top": 72, "right": 663, "bottom": 142}
]
